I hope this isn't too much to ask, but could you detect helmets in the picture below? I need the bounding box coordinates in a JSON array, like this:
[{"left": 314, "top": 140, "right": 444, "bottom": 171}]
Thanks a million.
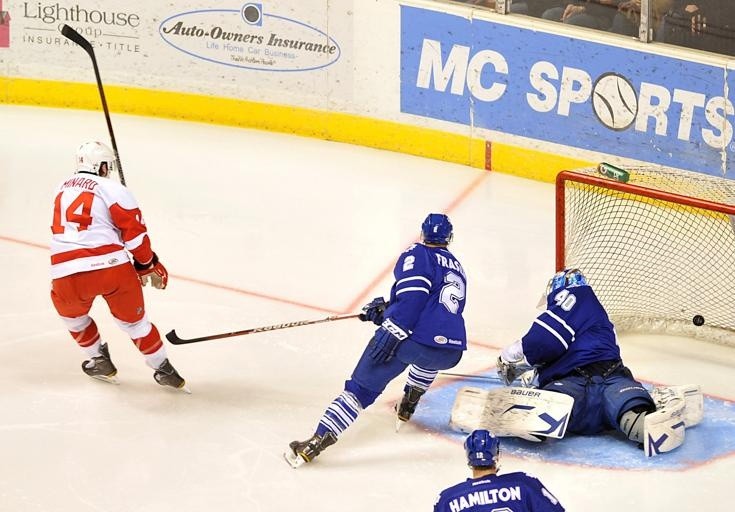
[
  {"left": 422, "top": 213, "right": 454, "bottom": 247},
  {"left": 545, "top": 268, "right": 588, "bottom": 291},
  {"left": 464, "top": 430, "right": 501, "bottom": 467},
  {"left": 75, "top": 140, "right": 114, "bottom": 174}
]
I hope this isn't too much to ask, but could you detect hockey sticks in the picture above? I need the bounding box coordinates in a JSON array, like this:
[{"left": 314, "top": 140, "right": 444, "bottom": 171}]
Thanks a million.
[
  {"left": 60, "top": 24, "right": 126, "bottom": 187},
  {"left": 165, "top": 313, "right": 368, "bottom": 344}
]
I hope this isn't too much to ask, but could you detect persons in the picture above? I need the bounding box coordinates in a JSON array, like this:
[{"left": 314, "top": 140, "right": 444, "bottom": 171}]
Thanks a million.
[
  {"left": 433, "top": 429, "right": 563, "bottom": 512},
  {"left": 290, "top": 213, "right": 467, "bottom": 468},
  {"left": 50, "top": 140, "right": 186, "bottom": 390},
  {"left": 542, "top": 0, "right": 616, "bottom": 31},
  {"left": 496, "top": 270, "right": 702, "bottom": 459}
]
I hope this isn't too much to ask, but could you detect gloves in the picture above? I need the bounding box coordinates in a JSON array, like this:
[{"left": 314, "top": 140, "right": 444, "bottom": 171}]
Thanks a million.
[
  {"left": 370, "top": 319, "right": 409, "bottom": 364},
  {"left": 496, "top": 341, "right": 532, "bottom": 385},
  {"left": 359, "top": 296, "right": 391, "bottom": 324},
  {"left": 133, "top": 255, "right": 169, "bottom": 288}
]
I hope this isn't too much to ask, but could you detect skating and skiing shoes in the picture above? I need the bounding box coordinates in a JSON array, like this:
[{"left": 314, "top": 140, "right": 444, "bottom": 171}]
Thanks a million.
[
  {"left": 82, "top": 344, "right": 120, "bottom": 384},
  {"left": 286, "top": 432, "right": 338, "bottom": 468},
  {"left": 153, "top": 361, "right": 192, "bottom": 394},
  {"left": 395, "top": 388, "right": 425, "bottom": 430}
]
